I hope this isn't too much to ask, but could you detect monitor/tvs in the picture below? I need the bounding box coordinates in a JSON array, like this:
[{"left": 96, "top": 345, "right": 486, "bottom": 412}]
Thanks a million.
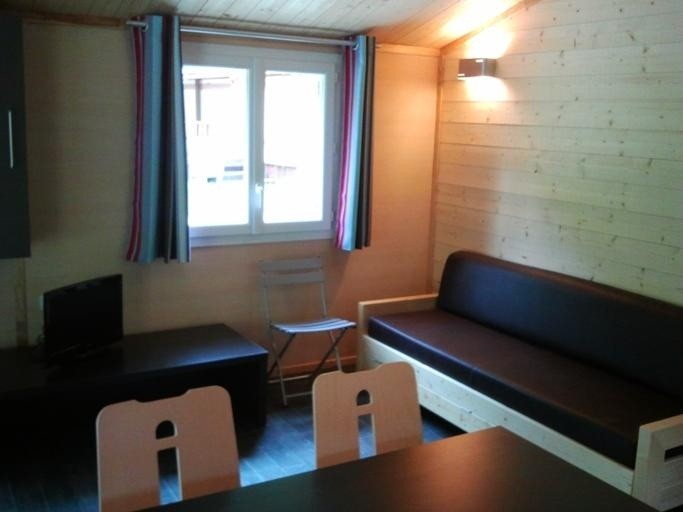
[{"left": 44, "top": 274, "right": 123, "bottom": 370}]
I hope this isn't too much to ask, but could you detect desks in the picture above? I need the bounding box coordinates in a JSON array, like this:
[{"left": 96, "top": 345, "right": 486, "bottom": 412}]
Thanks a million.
[
  {"left": 0, "top": 320, "right": 272, "bottom": 437},
  {"left": 129, "top": 424, "right": 659, "bottom": 512}
]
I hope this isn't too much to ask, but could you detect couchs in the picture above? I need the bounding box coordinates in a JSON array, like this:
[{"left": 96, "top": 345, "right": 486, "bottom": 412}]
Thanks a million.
[{"left": 352, "top": 249, "right": 683, "bottom": 510}]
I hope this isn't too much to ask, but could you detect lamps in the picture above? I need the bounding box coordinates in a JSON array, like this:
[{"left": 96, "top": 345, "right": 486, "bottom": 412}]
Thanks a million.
[{"left": 456, "top": 57, "right": 496, "bottom": 81}]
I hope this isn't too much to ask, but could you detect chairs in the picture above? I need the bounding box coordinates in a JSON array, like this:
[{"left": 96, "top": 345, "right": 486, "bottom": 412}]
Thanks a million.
[
  {"left": 92, "top": 361, "right": 422, "bottom": 512},
  {"left": 255, "top": 254, "right": 356, "bottom": 404}
]
